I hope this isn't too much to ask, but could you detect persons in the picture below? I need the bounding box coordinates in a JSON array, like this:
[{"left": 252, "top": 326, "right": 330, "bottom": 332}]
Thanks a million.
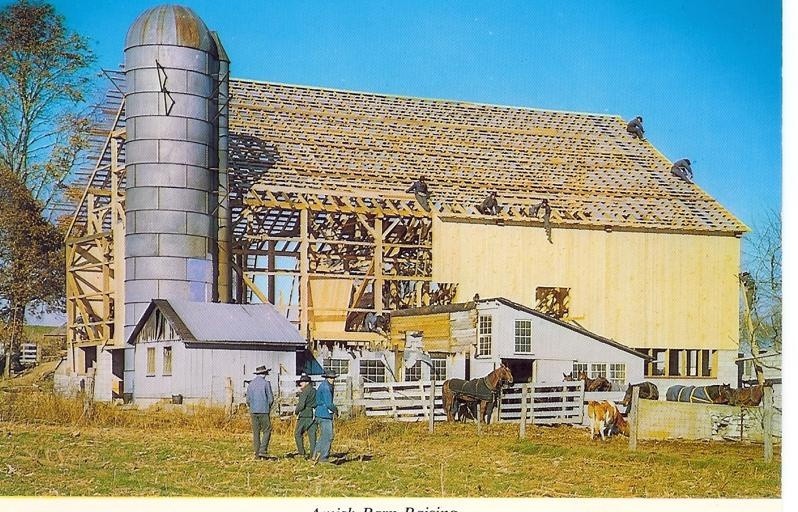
[
  {"left": 314, "top": 370, "right": 341, "bottom": 464},
  {"left": 72, "top": 302, "right": 114, "bottom": 340},
  {"left": 478, "top": 192, "right": 497, "bottom": 216},
  {"left": 531, "top": 200, "right": 552, "bottom": 236},
  {"left": 669, "top": 158, "right": 695, "bottom": 184},
  {"left": 405, "top": 175, "right": 430, "bottom": 212},
  {"left": 289, "top": 375, "right": 318, "bottom": 459},
  {"left": 738, "top": 271, "right": 759, "bottom": 311},
  {"left": 246, "top": 365, "right": 277, "bottom": 462},
  {"left": 364, "top": 313, "right": 392, "bottom": 341},
  {"left": 625, "top": 116, "right": 646, "bottom": 141}
]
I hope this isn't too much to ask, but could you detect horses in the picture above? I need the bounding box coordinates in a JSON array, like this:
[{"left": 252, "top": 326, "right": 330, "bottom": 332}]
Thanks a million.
[
  {"left": 666, "top": 382, "right": 735, "bottom": 405},
  {"left": 713, "top": 381, "right": 775, "bottom": 406},
  {"left": 589, "top": 399, "right": 627, "bottom": 441},
  {"left": 622, "top": 380, "right": 660, "bottom": 406},
  {"left": 442, "top": 363, "right": 514, "bottom": 424},
  {"left": 560, "top": 370, "right": 629, "bottom": 392}
]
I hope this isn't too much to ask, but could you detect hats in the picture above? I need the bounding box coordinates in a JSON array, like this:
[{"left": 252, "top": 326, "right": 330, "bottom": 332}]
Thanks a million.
[
  {"left": 252, "top": 365, "right": 270, "bottom": 374},
  {"left": 296, "top": 376, "right": 310, "bottom": 382},
  {"left": 320, "top": 371, "right": 339, "bottom": 378}
]
